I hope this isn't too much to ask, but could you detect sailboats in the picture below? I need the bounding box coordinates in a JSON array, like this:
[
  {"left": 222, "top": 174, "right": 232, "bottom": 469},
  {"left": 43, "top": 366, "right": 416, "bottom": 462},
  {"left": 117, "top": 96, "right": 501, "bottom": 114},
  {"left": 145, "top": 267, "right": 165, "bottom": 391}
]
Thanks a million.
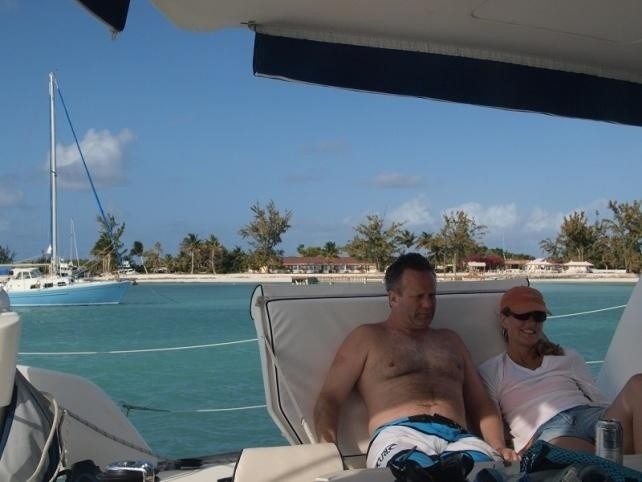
[{"left": 0, "top": 73, "right": 133, "bottom": 308}]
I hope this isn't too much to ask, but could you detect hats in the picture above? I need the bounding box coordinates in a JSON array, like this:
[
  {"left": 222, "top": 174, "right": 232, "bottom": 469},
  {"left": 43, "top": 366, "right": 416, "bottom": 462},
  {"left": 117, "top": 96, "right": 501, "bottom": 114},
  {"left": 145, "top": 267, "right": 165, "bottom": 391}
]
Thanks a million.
[{"left": 501, "top": 286, "right": 551, "bottom": 315}]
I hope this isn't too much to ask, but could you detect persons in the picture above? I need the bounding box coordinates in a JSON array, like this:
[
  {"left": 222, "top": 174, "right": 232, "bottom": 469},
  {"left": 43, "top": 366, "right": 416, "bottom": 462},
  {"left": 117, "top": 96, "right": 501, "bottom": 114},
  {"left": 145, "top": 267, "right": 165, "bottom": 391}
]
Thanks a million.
[
  {"left": 313, "top": 252, "right": 522, "bottom": 482},
  {"left": 477, "top": 286, "right": 642, "bottom": 458}
]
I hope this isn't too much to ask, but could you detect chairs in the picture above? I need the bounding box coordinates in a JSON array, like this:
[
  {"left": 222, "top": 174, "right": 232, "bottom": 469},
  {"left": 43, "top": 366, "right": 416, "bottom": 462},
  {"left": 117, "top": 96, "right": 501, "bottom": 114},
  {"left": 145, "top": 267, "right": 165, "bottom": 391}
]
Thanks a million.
[{"left": 249, "top": 278, "right": 642, "bottom": 482}]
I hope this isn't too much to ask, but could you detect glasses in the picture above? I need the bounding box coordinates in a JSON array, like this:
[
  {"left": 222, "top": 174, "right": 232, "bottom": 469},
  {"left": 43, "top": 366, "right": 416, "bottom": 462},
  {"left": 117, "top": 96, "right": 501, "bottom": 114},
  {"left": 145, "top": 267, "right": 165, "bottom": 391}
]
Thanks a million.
[{"left": 504, "top": 311, "right": 546, "bottom": 322}]
